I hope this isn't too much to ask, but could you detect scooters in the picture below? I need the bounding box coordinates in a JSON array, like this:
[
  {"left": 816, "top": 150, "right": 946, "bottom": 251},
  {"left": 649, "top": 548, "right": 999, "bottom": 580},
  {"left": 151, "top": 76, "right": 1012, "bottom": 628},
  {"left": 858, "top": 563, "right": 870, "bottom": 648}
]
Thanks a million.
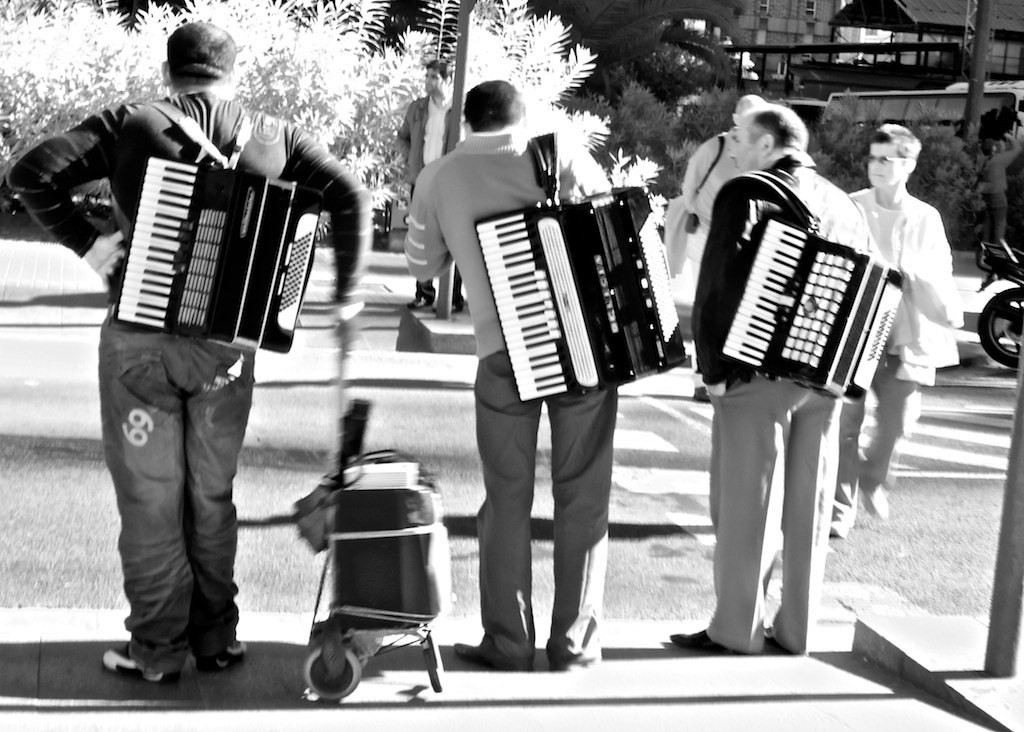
[{"left": 975, "top": 237, "right": 1024, "bottom": 369}]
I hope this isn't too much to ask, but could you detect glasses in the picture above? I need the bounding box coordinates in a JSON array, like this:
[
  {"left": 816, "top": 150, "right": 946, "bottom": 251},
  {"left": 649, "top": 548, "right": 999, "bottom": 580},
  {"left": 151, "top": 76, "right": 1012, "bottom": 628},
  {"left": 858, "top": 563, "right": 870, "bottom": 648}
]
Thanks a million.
[{"left": 865, "top": 153, "right": 912, "bottom": 165}]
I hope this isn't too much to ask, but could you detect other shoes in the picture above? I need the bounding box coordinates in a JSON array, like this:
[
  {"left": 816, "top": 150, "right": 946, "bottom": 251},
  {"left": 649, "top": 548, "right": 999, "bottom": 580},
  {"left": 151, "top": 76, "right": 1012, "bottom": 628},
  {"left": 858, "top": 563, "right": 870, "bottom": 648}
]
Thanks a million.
[
  {"left": 861, "top": 488, "right": 890, "bottom": 522},
  {"left": 764, "top": 626, "right": 801, "bottom": 655},
  {"left": 407, "top": 297, "right": 433, "bottom": 311},
  {"left": 669, "top": 629, "right": 756, "bottom": 656},
  {"left": 693, "top": 386, "right": 712, "bottom": 403},
  {"left": 431, "top": 303, "right": 463, "bottom": 313},
  {"left": 547, "top": 652, "right": 603, "bottom": 674},
  {"left": 829, "top": 520, "right": 849, "bottom": 538},
  {"left": 453, "top": 634, "right": 535, "bottom": 672}
]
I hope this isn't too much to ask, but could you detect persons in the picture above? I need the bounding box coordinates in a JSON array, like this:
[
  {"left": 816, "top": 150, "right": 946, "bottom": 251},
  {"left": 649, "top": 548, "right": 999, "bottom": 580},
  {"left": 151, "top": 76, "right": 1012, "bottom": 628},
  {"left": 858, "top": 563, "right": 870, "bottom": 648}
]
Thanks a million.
[
  {"left": 975, "top": 133, "right": 1020, "bottom": 247},
  {"left": 669, "top": 102, "right": 870, "bottom": 657},
  {"left": 391, "top": 57, "right": 470, "bottom": 317},
  {"left": 681, "top": 94, "right": 766, "bottom": 407},
  {"left": 828, "top": 125, "right": 965, "bottom": 538},
  {"left": 5, "top": 22, "right": 375, "bottom": 684},
  {"left": 401, "top": 79, "right": 620, "bottom": 675}
]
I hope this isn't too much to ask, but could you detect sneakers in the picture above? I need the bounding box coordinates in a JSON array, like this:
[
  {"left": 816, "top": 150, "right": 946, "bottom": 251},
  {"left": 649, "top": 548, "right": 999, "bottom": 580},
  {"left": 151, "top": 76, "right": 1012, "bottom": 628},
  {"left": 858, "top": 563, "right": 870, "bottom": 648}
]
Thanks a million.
[
  {"left": 195, "top": 641, "right": 247, "bottom": 672},
  {"left": 104, "top": 642, "right": 180, "bottom": 682}
]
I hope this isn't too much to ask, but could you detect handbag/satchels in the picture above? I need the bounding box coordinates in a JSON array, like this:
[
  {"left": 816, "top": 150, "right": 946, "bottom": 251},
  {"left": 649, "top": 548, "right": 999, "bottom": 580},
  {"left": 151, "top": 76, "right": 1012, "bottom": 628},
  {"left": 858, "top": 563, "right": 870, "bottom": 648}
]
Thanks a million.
[{"left": 663, "top": 195, "right": 699, "bottom": 279}]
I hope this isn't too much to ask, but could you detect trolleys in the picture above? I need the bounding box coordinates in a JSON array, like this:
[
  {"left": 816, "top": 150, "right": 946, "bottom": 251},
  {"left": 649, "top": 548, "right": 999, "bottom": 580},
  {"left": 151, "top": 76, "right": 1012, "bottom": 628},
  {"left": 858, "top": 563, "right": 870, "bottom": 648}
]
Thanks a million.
[{"left": 300, "top": 344, "right": 455, "bottom": 703}]
